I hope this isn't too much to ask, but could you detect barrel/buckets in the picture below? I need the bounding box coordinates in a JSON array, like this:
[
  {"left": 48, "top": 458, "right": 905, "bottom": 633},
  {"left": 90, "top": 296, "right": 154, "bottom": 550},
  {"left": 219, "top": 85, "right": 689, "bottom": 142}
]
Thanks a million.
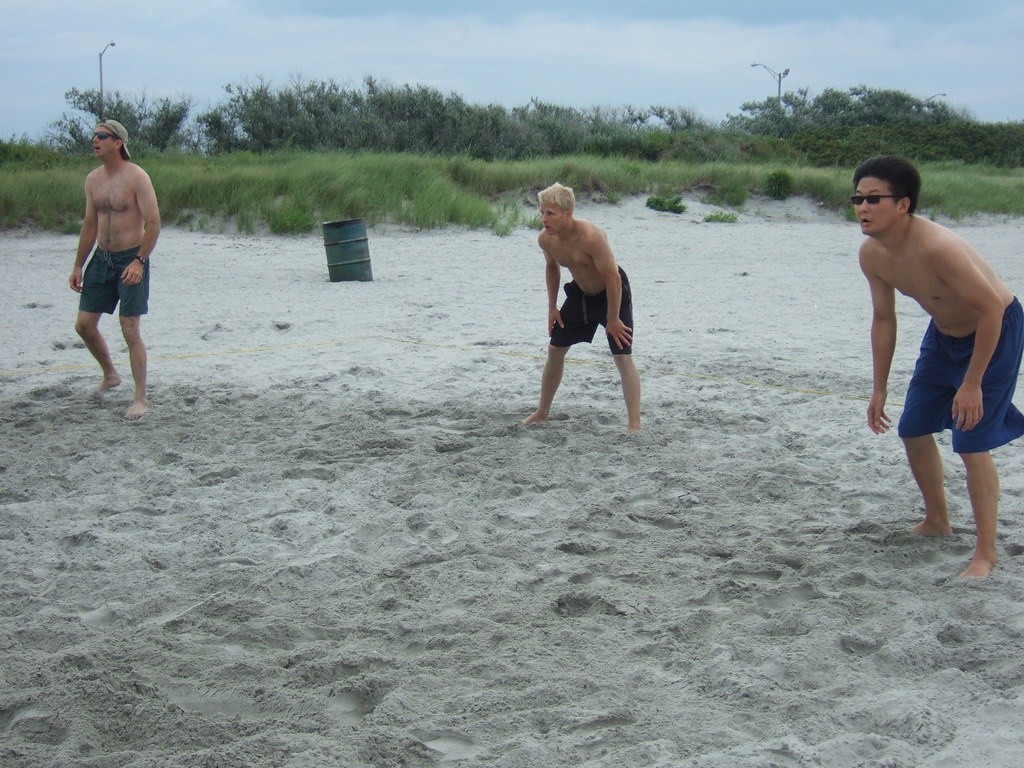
[{"left": 322, "top": 218, "right": 373, "bottom": 282}]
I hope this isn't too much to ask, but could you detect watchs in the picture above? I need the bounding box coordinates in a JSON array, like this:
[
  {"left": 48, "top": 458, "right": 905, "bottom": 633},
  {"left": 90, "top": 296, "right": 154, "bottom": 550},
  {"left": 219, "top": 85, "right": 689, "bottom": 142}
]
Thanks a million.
[{"left": 135, "top": 256, "right": 146, "bottom": 264}]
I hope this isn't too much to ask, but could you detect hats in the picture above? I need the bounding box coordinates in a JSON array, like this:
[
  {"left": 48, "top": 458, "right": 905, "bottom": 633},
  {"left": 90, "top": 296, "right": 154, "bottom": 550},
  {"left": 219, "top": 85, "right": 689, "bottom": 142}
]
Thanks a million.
[{"left": 98, "top": 119, "right": 131, "bottom": 161}]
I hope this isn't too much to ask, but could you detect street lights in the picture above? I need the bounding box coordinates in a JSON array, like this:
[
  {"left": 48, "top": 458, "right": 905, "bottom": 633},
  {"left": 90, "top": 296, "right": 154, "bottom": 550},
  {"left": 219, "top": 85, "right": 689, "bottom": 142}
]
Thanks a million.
[
  {"left": 99, "top": 41, "right": 115, "bottom": 119},
  {"left": 751, "top": 63, "right": 783, "bottom": 138}
]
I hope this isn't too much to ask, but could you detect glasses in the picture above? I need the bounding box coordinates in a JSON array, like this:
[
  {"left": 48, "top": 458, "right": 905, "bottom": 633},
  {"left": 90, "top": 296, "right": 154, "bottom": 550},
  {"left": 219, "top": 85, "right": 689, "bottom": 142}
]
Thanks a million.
[
  {"left": 92, "top": 131, "right": 119, "bottom": 139},
  {"left": 851, "top": 195, "right": 898, "bottom": 205}
]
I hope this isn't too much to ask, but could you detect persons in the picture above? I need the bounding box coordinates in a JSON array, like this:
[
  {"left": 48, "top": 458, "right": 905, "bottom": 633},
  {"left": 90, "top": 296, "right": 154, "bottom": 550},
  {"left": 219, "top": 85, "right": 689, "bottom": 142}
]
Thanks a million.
[
  {"left": 523, "top": 182, "right": 641, "bottom": 434},
  {"left": 69, "top": 119, "right": 161, "bottom": 421},
  {"left": 850, "top": 156, "right": 1023, "bottom": 579}
]
